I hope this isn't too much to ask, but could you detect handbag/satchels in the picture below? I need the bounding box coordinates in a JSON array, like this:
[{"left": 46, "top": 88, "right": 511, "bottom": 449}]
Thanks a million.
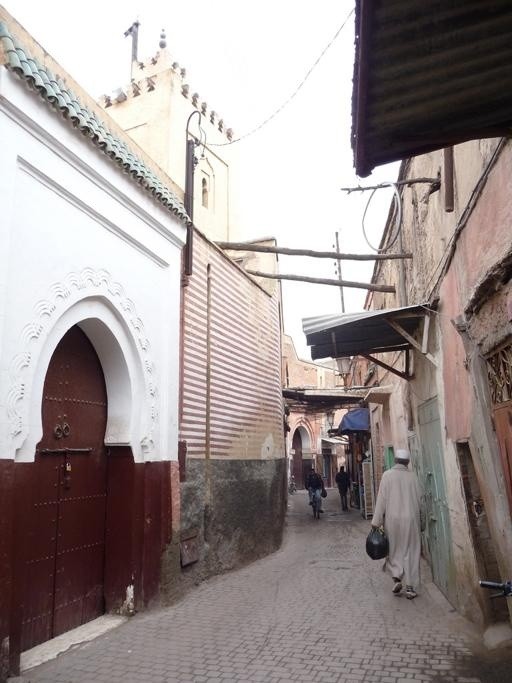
[
  {"left": 321, "top": 489, "right": 327, "bottom": 497},
  {"left": 366, "top": 526, "right": 389, "bottom": 560}
]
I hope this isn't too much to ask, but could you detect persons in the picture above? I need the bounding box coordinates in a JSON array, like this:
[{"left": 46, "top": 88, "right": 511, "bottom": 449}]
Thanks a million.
[
  {"left": 305, "top": 468, "right": 324, "bottom": 513},
  {"left": 335, "top": 466, "right": 352, "bottom": 512},
  {"left": 371, "top": 449, "right": 427, "bottom": 599}
]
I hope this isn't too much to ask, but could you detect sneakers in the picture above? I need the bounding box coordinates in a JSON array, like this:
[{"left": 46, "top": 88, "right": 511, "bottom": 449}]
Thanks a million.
[
  {"left": 392, "top": 582, "right": 402, "bottom": 593},
  {"left": 406, "top": 590, "right": 416, "bottom": 599}
]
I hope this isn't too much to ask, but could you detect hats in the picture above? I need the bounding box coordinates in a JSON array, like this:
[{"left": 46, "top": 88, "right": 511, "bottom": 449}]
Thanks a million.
[{"left": 395, "top": 449, "right": 409, "bottom": 459}]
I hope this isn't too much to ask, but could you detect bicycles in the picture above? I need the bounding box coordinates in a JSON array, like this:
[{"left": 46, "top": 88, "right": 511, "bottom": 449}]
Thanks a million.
[
  {"left": 309, "top": 486, "right": 324, "bottom": 519},
  {"left": 479, "top": 580, "right": 512, "bottom": 599}
]
